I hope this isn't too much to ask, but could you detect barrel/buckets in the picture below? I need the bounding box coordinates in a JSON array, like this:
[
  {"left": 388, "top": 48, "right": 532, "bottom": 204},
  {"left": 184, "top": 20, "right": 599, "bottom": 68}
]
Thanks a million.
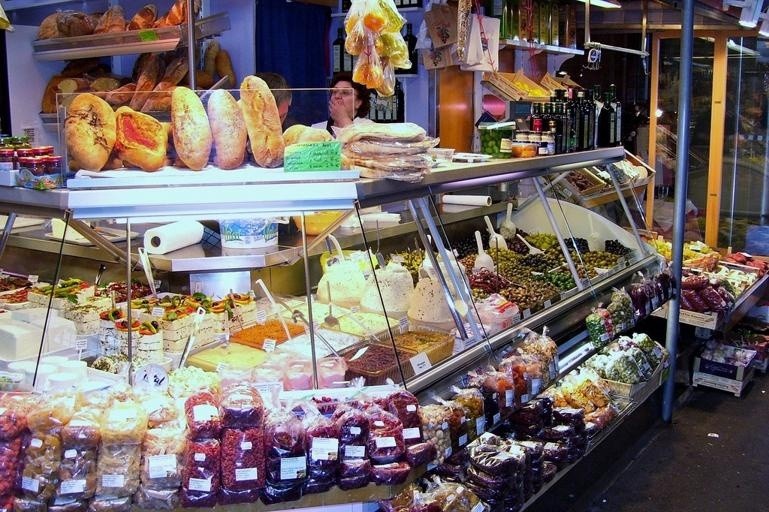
[
  {"left": 478, "top": 120, "right": 516, "bottom": 158},
  {"left": 220, "top": 218, "right": 278, "bottom": 251}
]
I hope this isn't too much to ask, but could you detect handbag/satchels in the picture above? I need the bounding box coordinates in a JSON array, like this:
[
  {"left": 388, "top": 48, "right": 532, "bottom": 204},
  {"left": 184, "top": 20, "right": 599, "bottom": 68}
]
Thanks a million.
[{"left": 422, "top": 3, "right": 501, "bottom": 72}]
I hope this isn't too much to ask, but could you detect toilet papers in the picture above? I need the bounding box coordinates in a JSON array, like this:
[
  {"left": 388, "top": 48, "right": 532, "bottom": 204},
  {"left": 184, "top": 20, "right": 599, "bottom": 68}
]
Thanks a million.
[
  {"left": 441, "top": 193, "right": 493, "bottom": 208},
  {"left": 144, "top": 221, "right": 205, "bottom": 255}
]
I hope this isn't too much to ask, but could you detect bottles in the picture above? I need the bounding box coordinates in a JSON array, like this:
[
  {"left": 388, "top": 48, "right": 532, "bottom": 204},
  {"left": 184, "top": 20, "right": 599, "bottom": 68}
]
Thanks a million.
[
  {"left": 528, "top": 82, "right": 624, "bottom": 152},
  {"left": 332, "top": 0, "right": 424, "bottom": 124}
]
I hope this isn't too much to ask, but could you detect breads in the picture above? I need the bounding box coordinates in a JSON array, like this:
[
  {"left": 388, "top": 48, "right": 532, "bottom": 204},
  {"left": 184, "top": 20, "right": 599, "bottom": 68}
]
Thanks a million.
[{"left": 37, "top": 1, "right": 350, "bottom": 170}]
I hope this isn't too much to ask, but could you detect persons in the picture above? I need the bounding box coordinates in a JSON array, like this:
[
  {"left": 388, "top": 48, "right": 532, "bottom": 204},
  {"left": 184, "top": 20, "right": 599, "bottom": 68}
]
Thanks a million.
[
  {"left": 308, "top": 74, "right": 378, "bottom": 141},
  {"left": 243, "top": 71, "right": 293, "bottom": 165}
]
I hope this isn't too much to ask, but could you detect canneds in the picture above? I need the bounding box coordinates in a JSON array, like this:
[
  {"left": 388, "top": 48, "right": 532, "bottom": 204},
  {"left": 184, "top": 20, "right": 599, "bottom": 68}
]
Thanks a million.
[
  {"left": 515, "top": 130, "right": 555, "bottom": 156},
  {"left": 0, "top": 146, "right": 62, "bottom": 176}
]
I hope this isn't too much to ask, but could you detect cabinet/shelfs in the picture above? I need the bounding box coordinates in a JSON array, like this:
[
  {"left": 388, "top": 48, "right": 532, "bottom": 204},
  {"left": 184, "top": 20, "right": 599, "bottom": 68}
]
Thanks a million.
[
  {"left": 0, "top": 145, "right": 677, "bottom": 468},
  {"left": 32, "top": 2, "right": 239, "bottom": 133}
]
